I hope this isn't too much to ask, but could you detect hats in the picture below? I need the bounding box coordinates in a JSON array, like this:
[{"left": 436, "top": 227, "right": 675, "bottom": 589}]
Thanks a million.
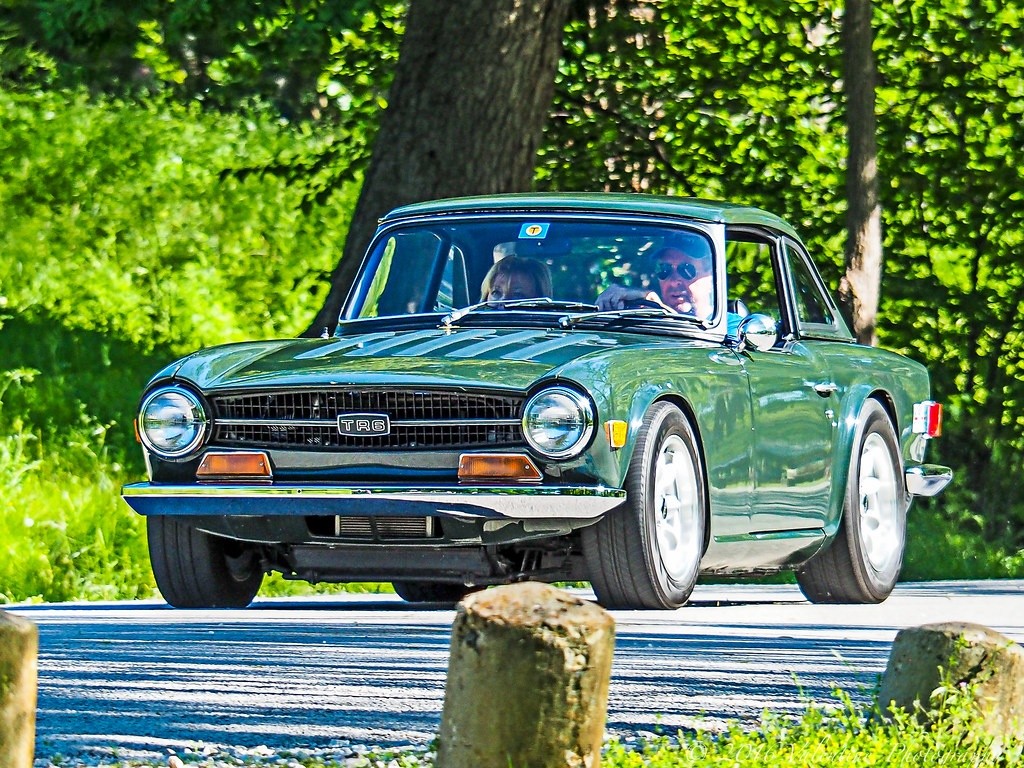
[{"left": 651, "top": 237, "right": 711, "bottom": 260}]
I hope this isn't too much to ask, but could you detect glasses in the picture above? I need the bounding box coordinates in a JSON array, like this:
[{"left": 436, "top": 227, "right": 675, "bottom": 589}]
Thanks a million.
[{"left": 654, "top": 263, "right": 707, "bottom": 280}]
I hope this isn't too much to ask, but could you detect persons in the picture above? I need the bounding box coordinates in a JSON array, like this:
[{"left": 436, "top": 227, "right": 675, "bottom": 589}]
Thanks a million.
[
  {"left": 478, "top": 254, "right": 552, "bottom": 309},
  {"left": 594, "top": 235, "right": 743, "bottom": 335}
]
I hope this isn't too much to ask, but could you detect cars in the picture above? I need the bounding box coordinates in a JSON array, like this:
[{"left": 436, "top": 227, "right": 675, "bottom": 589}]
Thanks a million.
[{"left": 119, "top": 191, "right": 951, "bottom": 612}]
